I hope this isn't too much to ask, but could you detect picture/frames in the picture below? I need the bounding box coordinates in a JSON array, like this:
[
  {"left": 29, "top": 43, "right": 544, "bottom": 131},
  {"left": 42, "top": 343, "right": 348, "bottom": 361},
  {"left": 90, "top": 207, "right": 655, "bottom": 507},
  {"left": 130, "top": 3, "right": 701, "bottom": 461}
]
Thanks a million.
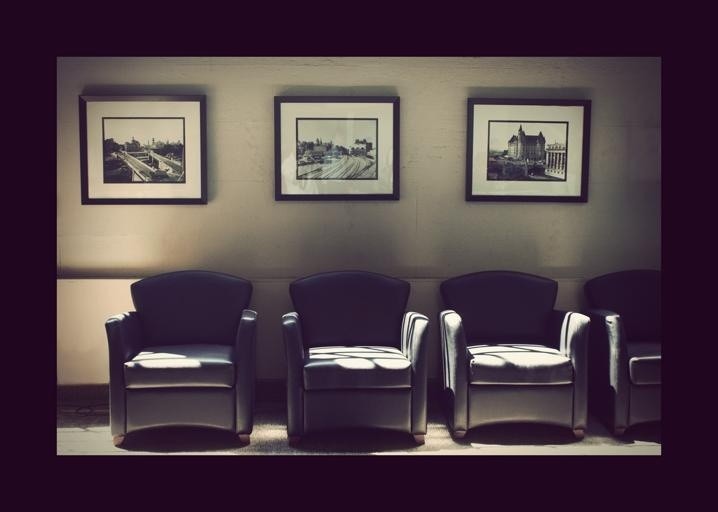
[
  {"left": 78, "top": 93, "right": 207, "bottom": 205},
  {"left": 466, "top": 97, "right": 591, "bottom": 202},
  {"left": 274, "top": 95, "right": 400, "bottom": 201}
]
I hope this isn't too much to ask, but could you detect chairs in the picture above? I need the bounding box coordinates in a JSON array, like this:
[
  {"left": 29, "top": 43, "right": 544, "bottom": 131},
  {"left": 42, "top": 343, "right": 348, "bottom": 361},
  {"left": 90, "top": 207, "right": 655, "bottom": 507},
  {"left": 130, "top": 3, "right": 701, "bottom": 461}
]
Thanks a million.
[
  {"left": 282, "top": 271, "right": 430, "bottom": 447},
  {"left": 438, "top": 271, "right": 591, "bottom": 442},
  {"left": 105, "top": 271, "right": 258, "bottom": 447},
  {"left": 584, "top": 269, "right": 662, "bottom": 438}
]
